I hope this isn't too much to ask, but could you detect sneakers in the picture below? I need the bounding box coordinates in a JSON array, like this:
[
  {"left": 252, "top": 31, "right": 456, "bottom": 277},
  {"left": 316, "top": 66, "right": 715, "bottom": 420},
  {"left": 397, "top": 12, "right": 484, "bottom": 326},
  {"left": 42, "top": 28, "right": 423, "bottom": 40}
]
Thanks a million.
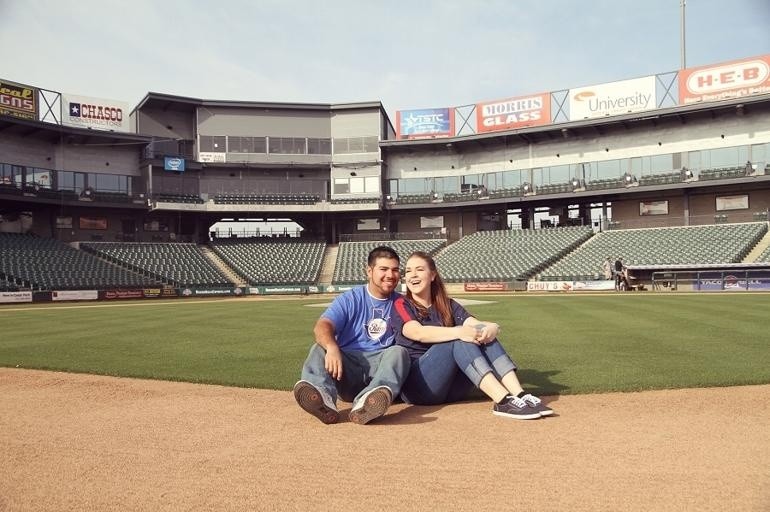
[
  {"left": 347, "top": 384, "right": 394, "bottom": 425},
  {"left": 292, "top": 379, "right": 338, "bottom": 425},
  {"left": 492, "top": 396, "right": 541, "bottom": 420},
  {"left": 519, "top": 393, "right": 554, "bottom": 416}
]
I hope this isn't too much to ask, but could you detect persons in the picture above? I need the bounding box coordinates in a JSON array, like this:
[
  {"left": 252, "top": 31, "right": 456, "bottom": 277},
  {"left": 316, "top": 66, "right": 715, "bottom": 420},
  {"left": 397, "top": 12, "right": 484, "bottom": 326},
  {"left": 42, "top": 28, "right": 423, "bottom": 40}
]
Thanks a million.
[
  {"left": 291, "top": 245, "right": 412, "bottom": 425},
  {"left": 390, "top": 250, "right": 555, "bottom": 419},
  {"left": 600, "top": 254, "right": 613, "bottom": 279},
  {"left": 615, "top": 257, "right": 631, "bottom": 287}
]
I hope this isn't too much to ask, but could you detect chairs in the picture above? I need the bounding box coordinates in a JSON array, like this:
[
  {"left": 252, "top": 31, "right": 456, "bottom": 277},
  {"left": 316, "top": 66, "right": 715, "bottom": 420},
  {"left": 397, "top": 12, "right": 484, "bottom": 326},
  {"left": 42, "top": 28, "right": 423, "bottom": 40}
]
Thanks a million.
[
  {"left": 332, "top": 223, "right": 746, "bottom": 290},
  {"left": 3, "top": 174, "right": 323, "bottom": 213},
  {"left": 335, "top": 161, "right": 746, "bottom": 205},
  {"left": 6, "top": 227, "right": 329, "bottom": 292}
]
